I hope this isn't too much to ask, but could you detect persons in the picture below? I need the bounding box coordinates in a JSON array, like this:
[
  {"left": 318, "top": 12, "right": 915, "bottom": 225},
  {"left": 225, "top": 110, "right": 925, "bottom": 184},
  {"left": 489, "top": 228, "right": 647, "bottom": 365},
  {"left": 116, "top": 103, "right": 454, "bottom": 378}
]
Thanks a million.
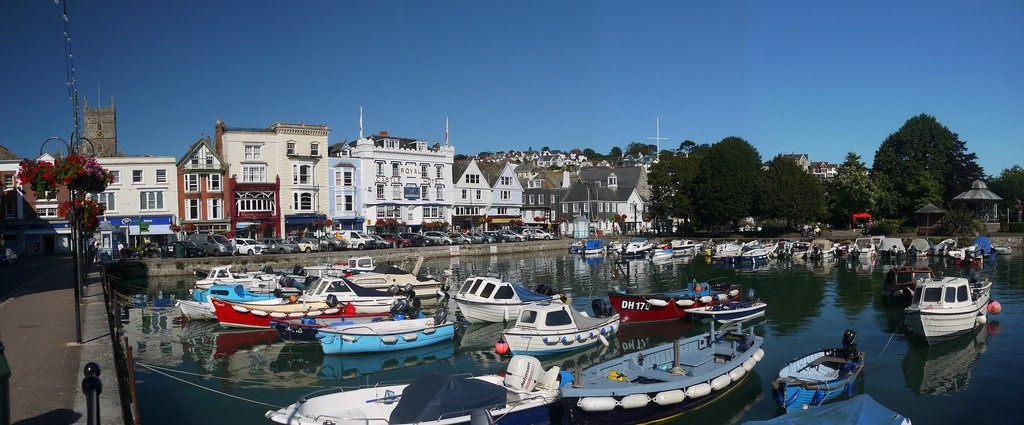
[
  {"left": 90, "top": 241, "right": 95, "bottom": 258},
  {"left": 463, "top": 228, "right": 469, "bottom": 234},
  {"left": 527, "top": 228, "right": 531, "bottom": 240},
  {"left": 814, "top": 226, "right": 821, "bottom": 235},
  {"left": 531, "top": 229, "right": 537, "bottom": 240},
  {"left": 118, "top": 242, "right": 123, "bottom": 251},
  {"left": 804, "top": 223, "right": 808, "bottom": 236}
]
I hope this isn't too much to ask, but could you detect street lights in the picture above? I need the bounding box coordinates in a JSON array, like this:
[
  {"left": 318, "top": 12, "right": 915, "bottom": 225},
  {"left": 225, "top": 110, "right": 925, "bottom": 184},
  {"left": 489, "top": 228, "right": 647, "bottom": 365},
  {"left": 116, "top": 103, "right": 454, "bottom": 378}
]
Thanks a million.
[
  {"left": 586, "top": 188, "right": 590, "bottom": 220},
  {"left": 633, "top": 202, "right": 638, "bottom": 236}
]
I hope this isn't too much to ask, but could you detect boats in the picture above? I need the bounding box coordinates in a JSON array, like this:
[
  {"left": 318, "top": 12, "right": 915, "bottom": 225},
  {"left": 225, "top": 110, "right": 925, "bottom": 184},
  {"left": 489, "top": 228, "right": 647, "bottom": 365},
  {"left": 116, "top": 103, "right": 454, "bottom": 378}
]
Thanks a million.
[
  {"left": 904, "top": 272, "right": 994, "bottom": 346},
  {"left": 740, "top": 389, "right": 911, "bottom": 425},
  {"left": 881, "top": 264, "right": 937, "bottom": 303},
  {"left": 451, "top": 274, "right": 563, "bottom": 324},
  {"left": 172, "top": 253, "right": 455, "bottom": 354},
  {"left": 771, "top": 329, "right": 866, "bottom": 413},
  {"left": 567, "top": 235, "right": 1014, "bottom": 274},
  {"left": 683, "top": 288, "right": 767, "bottom": 326},
  {"left": 264, "top": 354, "right": 576, "bottom": 425},
  {"left": 899, "top": 318, "right": 992, "bottom": 397},
  {"left": 556, "top": 321, "right": 766, "bottom": 425},
  {"left": 604, "top": 275, "right": 744, "bottom": 324},
  {"left": 498, "top": 297, "right": 621, "bottom": 358}
]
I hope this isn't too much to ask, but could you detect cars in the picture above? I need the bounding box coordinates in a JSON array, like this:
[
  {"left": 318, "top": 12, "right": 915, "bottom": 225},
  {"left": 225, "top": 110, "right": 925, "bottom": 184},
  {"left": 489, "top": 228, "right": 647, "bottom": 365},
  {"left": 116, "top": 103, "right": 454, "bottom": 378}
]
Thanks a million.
[
  {"left": 0, "top": 247, "right": 19, "bottom": 267},
  {"left": 167, "top": 225, "right": 556, "bottom": 258}
]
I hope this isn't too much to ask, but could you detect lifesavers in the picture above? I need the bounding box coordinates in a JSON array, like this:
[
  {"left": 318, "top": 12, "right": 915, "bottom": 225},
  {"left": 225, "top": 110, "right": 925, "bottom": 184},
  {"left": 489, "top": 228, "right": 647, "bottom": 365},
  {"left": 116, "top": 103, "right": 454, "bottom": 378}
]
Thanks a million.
[
  {"left": 681, "top": 241, "right": 684, "bottom": 244},
  {"left": 582, "top": 240, "right": 586, "bottom": 244}
]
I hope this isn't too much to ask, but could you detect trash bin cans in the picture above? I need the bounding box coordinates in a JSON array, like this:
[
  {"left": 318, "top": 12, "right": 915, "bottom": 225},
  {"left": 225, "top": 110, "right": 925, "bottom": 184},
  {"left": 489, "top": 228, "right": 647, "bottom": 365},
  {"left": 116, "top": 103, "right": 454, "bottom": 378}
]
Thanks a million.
[
  {"left": 0, "top": 342, "right": 12, "bottom": 425},
  {"left": 175, "top": 244, "right": 185, "bottom": 258}
]
